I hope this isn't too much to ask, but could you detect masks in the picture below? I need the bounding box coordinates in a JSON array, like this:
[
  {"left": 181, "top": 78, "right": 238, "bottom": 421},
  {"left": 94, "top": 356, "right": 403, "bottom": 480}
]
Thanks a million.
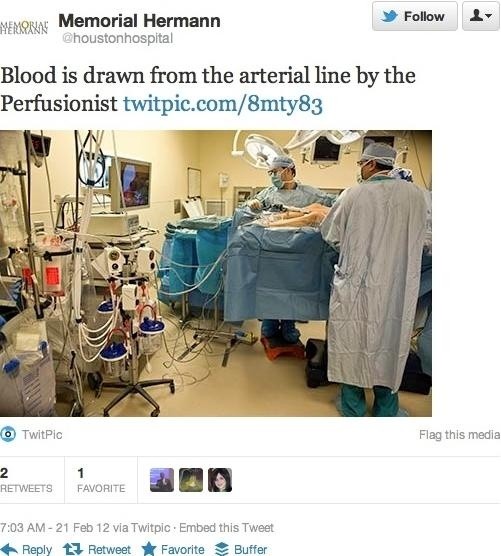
[
  {"left": 355, "top": 160, "right": 371, "bottom": 185},
  {"left": 271, "top": 169, "right": 286, "bottom": 189}
]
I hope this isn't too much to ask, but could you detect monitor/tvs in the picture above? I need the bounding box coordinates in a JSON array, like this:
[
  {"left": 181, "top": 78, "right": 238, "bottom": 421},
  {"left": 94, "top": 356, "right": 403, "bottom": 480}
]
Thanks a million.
[{"left": 111, "top": 157, "right": 152, "bottom": 211}]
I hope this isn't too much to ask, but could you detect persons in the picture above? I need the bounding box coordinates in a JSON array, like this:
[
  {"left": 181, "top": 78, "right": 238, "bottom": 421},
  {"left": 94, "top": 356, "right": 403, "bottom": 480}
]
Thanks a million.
[
  {"left": 318, "top": 142, "right": 432, "bottom": 417},
  {"left": 208, "top": 468, "right": 232, "bottom": 492},
  {"left": 156, "top": 473, "right": 172, "bottom": 492},
  {"left": 242, "top": 157, "right": 339, "bottom": 342}
]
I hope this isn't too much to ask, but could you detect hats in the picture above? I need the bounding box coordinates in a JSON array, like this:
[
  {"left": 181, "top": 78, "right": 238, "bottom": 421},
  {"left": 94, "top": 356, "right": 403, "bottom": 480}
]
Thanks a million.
[
  {"left": 269, "top": 155, "right": 295, "bottom": 170},
  {"left": 360, "top": 142, "right": 397, "bottom": 166}
]
